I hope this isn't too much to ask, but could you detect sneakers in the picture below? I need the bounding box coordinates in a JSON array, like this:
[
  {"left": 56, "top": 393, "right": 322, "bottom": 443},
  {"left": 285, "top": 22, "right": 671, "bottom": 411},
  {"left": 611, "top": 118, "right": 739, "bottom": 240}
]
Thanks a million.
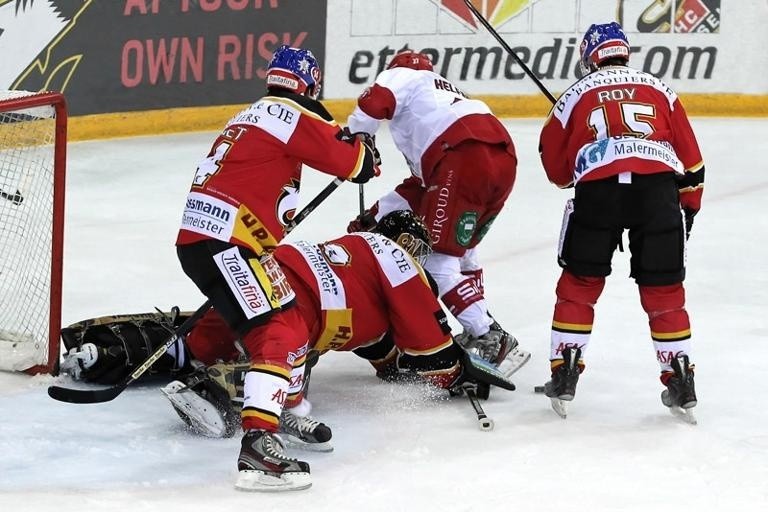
[
  {"left": 456, "top": 309, "right": 696, "bottom": 408},
  {"left": 63, "top": 344, "right": 333, "bottom": 475}
]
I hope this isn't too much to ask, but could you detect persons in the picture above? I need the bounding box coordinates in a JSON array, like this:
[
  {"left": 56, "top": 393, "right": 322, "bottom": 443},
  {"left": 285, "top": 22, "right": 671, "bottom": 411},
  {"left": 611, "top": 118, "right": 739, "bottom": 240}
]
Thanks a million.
[
  {"left": 539, "top": 22, "right": 705, "bottom": 425},
  {"left": 176, "top": 47, "right": 380, "bottom": 493},
  {"left": 344, "top": 51, "right": 531, "bottom": 396},
  {"left": 58, "top": 210, "right": 491, "bottom": 438}
]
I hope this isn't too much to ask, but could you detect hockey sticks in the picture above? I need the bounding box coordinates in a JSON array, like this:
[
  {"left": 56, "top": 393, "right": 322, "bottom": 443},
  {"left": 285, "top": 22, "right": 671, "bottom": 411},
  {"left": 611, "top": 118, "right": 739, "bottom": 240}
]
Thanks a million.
[{"left": 47, "top": 177, "right": 345, "bottom": 403}]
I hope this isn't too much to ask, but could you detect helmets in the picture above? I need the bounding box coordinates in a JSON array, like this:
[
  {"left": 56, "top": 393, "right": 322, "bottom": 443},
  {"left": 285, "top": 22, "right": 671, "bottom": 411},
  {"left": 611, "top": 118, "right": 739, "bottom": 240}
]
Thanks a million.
[
  {"left": 579, "top": 23, "right": 631, "bottom": 68},
  {"left": 267, "top": 45, "right": 322, "bottom": 101},
  {"left": 387, "top": 53, "right": 432, "bottom": 73},
  {"left": 379, "top": 212, "right": 433, "bottom": 268}
]
